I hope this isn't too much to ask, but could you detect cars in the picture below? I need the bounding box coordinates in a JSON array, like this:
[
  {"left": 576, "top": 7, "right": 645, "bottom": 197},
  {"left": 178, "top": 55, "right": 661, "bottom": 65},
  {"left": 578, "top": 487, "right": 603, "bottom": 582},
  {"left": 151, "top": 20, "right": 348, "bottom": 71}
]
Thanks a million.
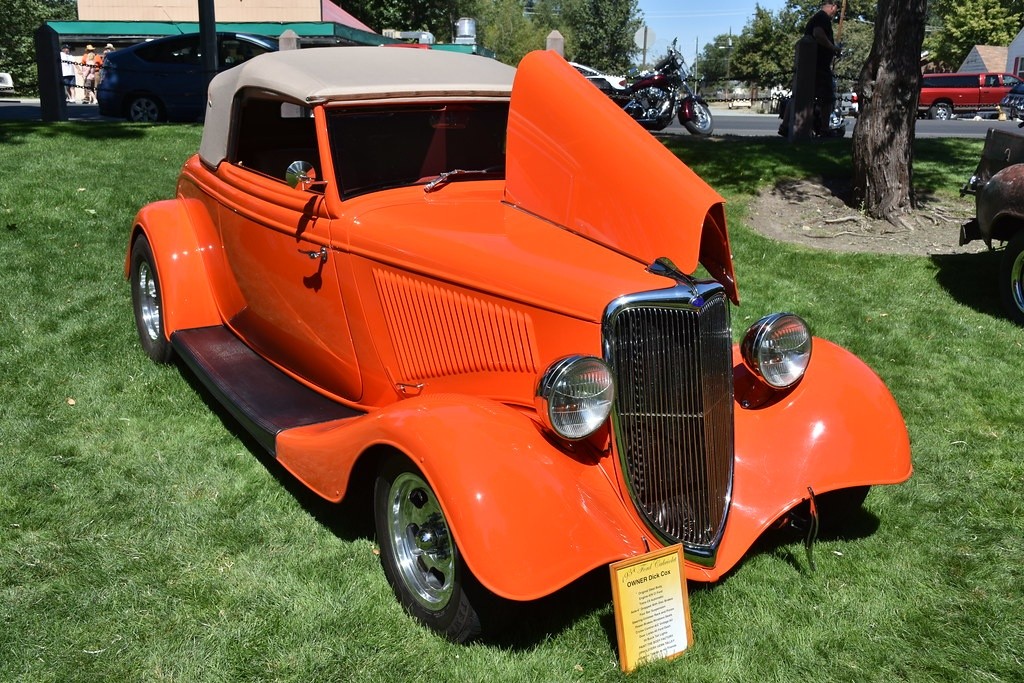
[
  {"left": 954, "top": 118, "right": 1024, "bottom": 322},
  {"left": 836, "top": 83, "right": 861, "bottom": 118},
  {"left": 92, "top": 32, "right": 282, "bottom": 126},
  {"left": 568, "top": 59, "right": 630, "bottom": 92},
  {"left": 128, "top": 36, "right": 914, "bottom": 645}
]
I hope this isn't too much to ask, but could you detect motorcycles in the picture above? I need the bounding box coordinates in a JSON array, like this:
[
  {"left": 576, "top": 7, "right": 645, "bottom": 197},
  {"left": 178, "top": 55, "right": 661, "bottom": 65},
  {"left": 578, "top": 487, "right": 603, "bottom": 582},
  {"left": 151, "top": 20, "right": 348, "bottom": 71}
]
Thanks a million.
[{"left": 588, "top": 37, "right": 716, "bottom": 135}]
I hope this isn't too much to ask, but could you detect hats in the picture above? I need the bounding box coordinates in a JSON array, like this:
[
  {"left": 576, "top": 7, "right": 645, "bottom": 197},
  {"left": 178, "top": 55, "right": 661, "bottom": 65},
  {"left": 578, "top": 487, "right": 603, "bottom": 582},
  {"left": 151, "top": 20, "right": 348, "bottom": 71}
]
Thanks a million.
[
  {"left": 62, "top": 45, "right": 71, "bottom": 49},
  {"left": 105, "top": 43, "right": 115, "bottom": 50},
  {"left": 85, "top": 45, "right": 96, "bottom": 50}
]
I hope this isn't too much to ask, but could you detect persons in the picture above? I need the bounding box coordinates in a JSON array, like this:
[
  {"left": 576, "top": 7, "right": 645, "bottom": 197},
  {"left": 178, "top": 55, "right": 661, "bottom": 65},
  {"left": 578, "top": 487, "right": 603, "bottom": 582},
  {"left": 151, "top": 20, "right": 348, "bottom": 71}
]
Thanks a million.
[
  {"left": 59, "top": 44, "right": 116, "bottom": 105},
  {"left": 777, "top": 0, "right": 841, "bottom": 137}
]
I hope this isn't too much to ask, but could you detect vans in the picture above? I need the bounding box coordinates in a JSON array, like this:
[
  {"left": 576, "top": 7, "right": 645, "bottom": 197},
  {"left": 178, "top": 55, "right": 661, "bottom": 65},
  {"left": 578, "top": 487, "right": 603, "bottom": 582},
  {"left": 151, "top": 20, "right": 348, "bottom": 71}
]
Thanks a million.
[{"left": 918, "top": 72, "right": 1024, "bottom": 121}]
